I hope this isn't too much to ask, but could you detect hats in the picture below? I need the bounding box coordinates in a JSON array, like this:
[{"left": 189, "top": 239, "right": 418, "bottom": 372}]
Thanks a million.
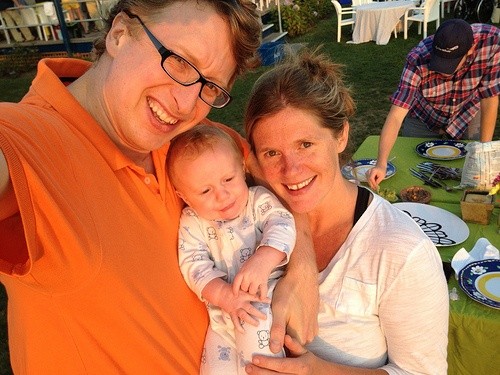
[{"left": 428, "top": 19, "right": 473, "bottom": 74}]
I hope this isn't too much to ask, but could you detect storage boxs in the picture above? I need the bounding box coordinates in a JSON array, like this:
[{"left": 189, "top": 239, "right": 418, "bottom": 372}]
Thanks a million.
[{"left": 257, "top": 41, "right": 283, "bottom": 66}]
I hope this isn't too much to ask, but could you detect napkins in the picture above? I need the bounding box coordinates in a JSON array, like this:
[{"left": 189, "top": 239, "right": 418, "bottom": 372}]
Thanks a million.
[{"left": 451, "top": 237, "right": 500, "bottom": 280}]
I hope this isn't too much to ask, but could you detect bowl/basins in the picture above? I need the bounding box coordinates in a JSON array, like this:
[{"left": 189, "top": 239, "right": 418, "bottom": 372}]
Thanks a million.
[
  {"left": 370, "top": 186, "right": 399, "bottom": 204},
  {"left": 400, "top": 186, "right": 431, "bottom": 204}
]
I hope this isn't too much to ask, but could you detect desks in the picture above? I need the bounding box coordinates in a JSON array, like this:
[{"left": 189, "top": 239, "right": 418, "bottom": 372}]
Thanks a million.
[
  {"left": 389, "top": 199, "right": 500, "bottom": 375},
  {"left": 349, "top": 135, "right": 500, "bottom": 208},
  {"left": 346, "top": 1, "right": 416, "bottom": 46},
  {"left": 21, "top": 2, "right": 59, "bottom": 26}
]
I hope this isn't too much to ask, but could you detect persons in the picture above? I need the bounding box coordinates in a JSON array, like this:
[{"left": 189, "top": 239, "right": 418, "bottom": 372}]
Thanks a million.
[
  {"left": 365, "top": 19, "right": 500, "bottom": 191},
  {"left": 165, "top": 124, "right": 297, "bottom": 375},
  {"left": 245, "top": 42, "right": 450, "bottom": 374},
  {"left": 1, "top": 0, "right": 319, "bottom": 374}
]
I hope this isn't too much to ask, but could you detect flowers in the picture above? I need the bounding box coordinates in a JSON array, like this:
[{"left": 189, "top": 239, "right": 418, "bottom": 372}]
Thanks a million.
[{"left": 486, "top": 172, "right": 500, "bottom": 196}]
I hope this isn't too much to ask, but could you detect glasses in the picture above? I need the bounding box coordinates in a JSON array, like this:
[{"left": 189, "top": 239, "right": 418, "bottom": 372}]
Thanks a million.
[{"left": 123, "top": 7, "right": 232, "bottom": 110}]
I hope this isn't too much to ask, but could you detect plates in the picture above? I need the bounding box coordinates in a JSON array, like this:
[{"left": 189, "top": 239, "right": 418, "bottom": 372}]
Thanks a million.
[
  {"left": 340, "top": 158, "right": 398, "bottom": 183},
  {"left": 458, "top": 259, "right": 500, "bottom": 310},
  {"left": 390, "top": 202, "right": 469, "bottom": 246},
  {"left": 414, "top": 140, "right": 469, "bottom": 161}
]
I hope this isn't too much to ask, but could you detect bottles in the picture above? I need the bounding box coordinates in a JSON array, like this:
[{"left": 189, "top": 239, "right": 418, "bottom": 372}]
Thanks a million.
[{"left": 47, "top": 10, "right": 85, "bottom": 41}]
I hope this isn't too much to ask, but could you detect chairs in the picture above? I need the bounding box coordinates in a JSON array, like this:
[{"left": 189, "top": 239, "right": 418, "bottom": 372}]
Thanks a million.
[
  {"left": 331, "top": 0, "right": 357, "bottom": 42},
  {"left": 351, "top": 0, "right": 373, "bottom": 30},
  {"left": 403, "top": 0, "right": 440, "bottom": 40}
]
